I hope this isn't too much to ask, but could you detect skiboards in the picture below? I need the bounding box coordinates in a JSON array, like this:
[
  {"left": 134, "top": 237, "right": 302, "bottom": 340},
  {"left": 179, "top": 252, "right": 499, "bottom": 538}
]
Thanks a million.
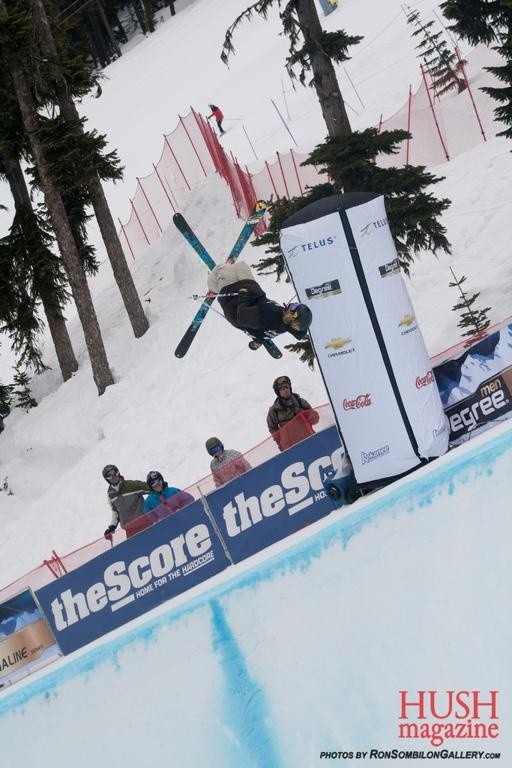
[{"left": 172, "top": 200, "right": 284, "bottom": 358}]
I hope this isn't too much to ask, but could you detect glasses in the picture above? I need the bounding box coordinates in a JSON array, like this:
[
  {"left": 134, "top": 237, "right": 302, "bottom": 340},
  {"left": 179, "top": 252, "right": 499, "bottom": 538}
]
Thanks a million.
[
  {"left": 289, "top": 304, "right": 299, "bottom": 311},
  {"left": 149, "top": 477, "right": 162, "bottom": 487},
  {"left": 278, "top": 384, "right": 289, "bottom": 391},
  {"left": 209, "top": 444, "right": 221, "bottom": 455}
]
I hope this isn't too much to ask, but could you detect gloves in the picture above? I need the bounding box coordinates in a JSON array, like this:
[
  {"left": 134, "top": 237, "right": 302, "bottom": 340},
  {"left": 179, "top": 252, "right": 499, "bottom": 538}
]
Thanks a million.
[
  {"left": 105, "top": 526, "right": 116, "bottom": 539},
  {"left": 239, "top": 287, "right": 252, "bottom": 295},
  {"left": 249, "top": 341, "right": 262, "bottom": 350}
]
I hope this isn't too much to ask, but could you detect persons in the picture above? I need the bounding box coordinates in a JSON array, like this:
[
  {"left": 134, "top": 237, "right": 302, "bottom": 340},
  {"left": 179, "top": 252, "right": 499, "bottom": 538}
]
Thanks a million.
[
  {"left": 204, "top": 435, "right": 252, "bottom": 487},
  {"left": 206, "top": 260, "right": 313, "bottom": 351},
  {"left": 207, "top": 103, "right": 226, "bottom": 134},
  {"left": 143, "top": 470, "right": 193, "bottom": 526},
  {"left": 264, "top": 374, "right": 321, "bottom": 453},
  {"left": 101, "top": 463, "right": 152, "bottom": 539}
]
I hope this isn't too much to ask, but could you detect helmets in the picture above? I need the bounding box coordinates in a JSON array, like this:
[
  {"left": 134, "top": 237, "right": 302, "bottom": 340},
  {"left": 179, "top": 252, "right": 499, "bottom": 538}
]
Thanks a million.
[
  {"left": 283, "top": 304, "right": 312, "bottom": 332},
  {"left": 273, "top": 376, "right": 292, "bottom": 397},
  {"left": 147, "top": 471, "right": 164, "bottom": 490},
  {"left": 206, "top": 438, "right": 224, "bottom": 457},
  {"left": 103, "top": 465, "right": 121, "bottom": 485}
]
